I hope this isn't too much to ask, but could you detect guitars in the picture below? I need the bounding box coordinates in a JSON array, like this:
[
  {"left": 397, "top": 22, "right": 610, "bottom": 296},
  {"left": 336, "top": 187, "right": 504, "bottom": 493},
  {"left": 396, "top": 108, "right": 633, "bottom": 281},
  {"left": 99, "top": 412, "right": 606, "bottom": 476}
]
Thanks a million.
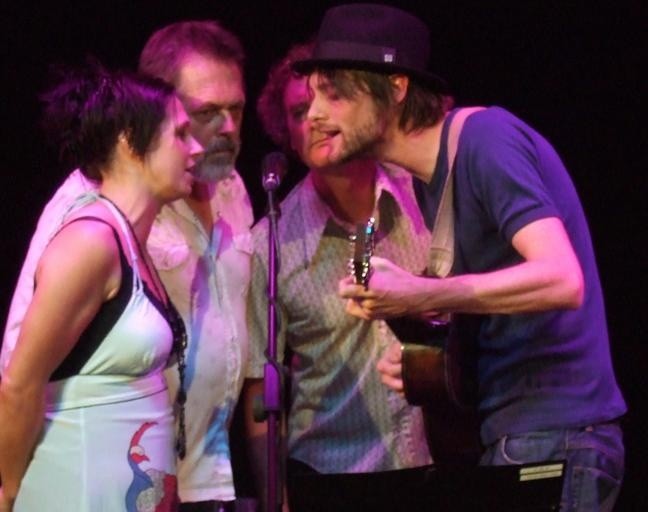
[{"left": 350, "top": 217, "right": 493, "bottom": 479}]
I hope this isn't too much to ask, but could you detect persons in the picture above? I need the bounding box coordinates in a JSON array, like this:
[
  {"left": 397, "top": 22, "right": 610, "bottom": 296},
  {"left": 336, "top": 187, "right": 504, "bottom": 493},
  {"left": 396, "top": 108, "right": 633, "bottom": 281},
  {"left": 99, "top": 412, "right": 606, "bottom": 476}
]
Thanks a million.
[
  {"left": 1, "top": 68, "right": 204, "bottom": 511},
  {"left": 243, "top": 43, "right": 433, "bottom": 511},
  {"left": 0, "top": 20, "right": 254, "bottom": 510},
  {"left": 289, "top": 0, "right": 630, "bottom": 512}
]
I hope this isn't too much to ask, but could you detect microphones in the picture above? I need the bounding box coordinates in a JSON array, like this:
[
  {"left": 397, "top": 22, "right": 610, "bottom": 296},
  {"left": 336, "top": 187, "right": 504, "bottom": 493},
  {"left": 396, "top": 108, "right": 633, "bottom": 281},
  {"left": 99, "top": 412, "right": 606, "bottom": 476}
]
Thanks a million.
[{"left": 260, "top": 151, "right": 289, "bottom": 194}]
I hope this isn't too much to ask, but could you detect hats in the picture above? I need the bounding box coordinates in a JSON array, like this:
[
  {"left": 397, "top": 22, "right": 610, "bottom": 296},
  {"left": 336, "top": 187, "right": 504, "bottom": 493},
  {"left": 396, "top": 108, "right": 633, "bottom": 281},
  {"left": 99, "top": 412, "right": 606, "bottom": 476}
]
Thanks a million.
[{"left": 293, "top": 3, "right": 452, "bottom": 88}]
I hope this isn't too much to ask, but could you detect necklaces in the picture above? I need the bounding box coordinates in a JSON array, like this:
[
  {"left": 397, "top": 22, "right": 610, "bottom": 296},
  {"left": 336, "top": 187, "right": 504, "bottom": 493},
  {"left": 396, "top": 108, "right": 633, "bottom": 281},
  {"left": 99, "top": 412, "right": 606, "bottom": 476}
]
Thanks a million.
[{"left": 98, "top": 194, "right": 188, "bottom": 459}]
